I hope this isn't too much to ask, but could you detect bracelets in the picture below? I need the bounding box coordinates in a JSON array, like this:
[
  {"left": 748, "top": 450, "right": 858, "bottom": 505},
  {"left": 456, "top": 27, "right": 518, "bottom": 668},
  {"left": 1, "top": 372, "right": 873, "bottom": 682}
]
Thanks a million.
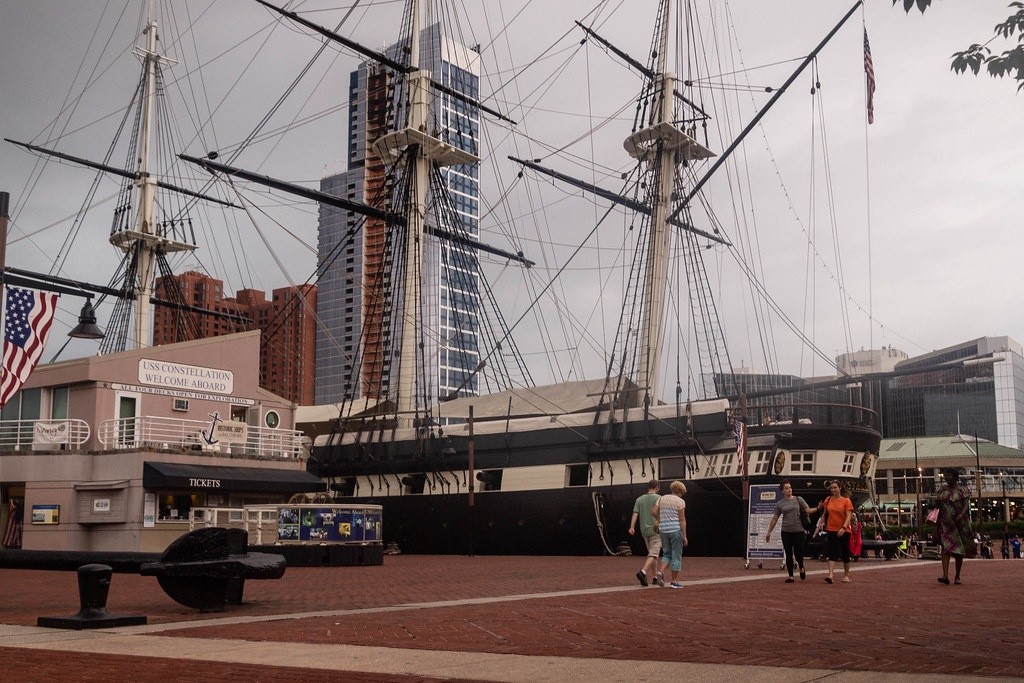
[
  {"left": 817, "top": 506, "right": 819, "bottom": 510},
  {"left": 841, "top": 527, "right": 846, "bottom": 530}
]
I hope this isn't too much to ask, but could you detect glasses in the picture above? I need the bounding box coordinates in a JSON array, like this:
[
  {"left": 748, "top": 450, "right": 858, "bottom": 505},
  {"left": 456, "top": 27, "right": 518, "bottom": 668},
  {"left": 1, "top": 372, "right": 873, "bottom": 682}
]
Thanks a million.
[{"left": 830, "top": 480, "right": 837, "bottom": 483}]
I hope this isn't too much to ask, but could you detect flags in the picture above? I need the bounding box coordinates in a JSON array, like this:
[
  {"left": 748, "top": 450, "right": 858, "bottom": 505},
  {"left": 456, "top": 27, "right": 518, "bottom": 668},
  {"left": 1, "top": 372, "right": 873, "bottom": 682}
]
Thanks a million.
[
  {"left": 735, "top": 421, "right": 744, "bottom": 467},
  {"left": 863, "top": 29, "right": 875, "bottom": 124},
  {"left": 0, "top": 286, "right": 57, "bottom": 409}
]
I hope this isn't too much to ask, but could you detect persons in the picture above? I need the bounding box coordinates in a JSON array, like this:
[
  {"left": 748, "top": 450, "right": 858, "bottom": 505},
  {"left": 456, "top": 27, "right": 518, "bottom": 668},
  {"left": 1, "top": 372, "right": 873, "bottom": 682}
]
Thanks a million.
[
  {"left": 813, "top": 509, "right": 863, "bottom": 562},
  {"left": 896, "top": 532, "right": 919, "bottom": 559},
  {"left": 651, "top": 481, "right": 688, "bottom": 589},
  {"left": 629, "top": 479, "right": 662, "bottom": 587},
  {"left": 975, "top": 531, "right": 1022, "bottom": 559},
  {"left": 819, "top": 480, "right": 854, "bottom": 584},
  {"left": 933, "top": 468, "right": 977, "bottom": 585},
  {"left": 876, "top": 532, "right": 883, "bottom": 558},
  {"left": 766, "top": 482, "right": 823, "bottom": 583}
]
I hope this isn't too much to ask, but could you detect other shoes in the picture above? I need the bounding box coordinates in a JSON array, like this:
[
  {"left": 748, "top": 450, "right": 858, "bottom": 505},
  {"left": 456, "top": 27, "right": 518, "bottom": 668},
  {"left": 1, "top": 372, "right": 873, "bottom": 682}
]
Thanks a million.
[
  {"left": 636, "top": 570, "right": 648, "bottom": 586},
  {"left": 670, "top": 582, "right": 684, "bottom": 588},
  {"left": 954, "top": 578, "right": 961, "bottom": 584},
  {"left": 657, "top": 570, "right": 665, "bottom": 586},
  {"left": 938, "top": 576, "right": 950, "bottom": 584},
  {"left": 800, "top": 566, "right": 806, "bottom": 581},
  {"left": 825, "top": 576, "right": 834, "bottom": 583},
  {"left": 842, "top": 576, "right": 851, "bottom": 583},
  {"left": 653, "top": 577, "right": 657, "bottom": 584},
  {"left": 785, "top": 577, "right": 794, "bottom": 583}
]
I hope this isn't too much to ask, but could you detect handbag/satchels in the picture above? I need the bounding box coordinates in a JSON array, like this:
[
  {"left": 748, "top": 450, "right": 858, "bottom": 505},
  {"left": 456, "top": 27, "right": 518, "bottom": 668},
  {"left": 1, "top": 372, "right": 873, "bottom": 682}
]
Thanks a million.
[
  {"left": 797, "top": 496, "right": 811, "bottom": 531},
  {"left": 823, "top": 495, "right": 831, "bottom": 532},
  {"left": 926, "top": 487, "right": 945, "bottom": 526}
]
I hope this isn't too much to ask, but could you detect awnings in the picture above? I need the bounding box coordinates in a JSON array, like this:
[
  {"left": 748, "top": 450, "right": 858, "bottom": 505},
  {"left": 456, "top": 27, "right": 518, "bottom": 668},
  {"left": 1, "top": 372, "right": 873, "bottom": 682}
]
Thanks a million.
[{"left": 143, "top": 461, "right": 327, "bottom": 494}]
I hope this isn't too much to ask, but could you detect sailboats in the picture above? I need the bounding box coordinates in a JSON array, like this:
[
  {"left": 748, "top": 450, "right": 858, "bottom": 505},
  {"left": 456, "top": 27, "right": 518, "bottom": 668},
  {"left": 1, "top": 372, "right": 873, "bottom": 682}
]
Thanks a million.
[{"left": 1, "top": 0, "right": 887, "bottom": 571}]
